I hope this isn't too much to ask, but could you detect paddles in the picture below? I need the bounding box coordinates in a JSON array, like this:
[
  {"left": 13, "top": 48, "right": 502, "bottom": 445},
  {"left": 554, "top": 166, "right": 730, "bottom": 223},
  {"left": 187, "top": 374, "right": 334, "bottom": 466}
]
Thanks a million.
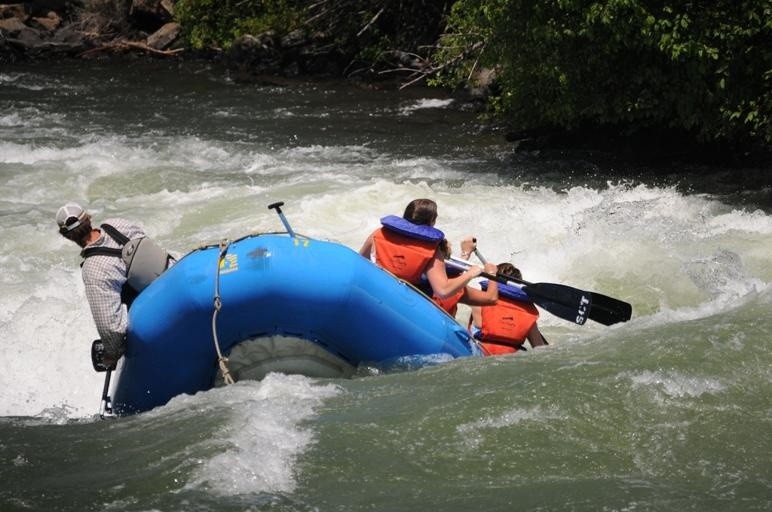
[
  {"left": 444, "top": 258, "right": 592, "bottom": 325},
  {"left": 452, "top": 259, "right": 632, "bottom": 327},
  {"left": 91, "top": 338, "right": 120, "bottom": 372}
]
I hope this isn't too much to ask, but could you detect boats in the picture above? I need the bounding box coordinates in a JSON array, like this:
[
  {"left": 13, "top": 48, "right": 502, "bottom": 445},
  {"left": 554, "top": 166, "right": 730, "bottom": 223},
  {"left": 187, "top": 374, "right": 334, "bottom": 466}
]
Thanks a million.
[{"left": 111, "top": 232, "right": 483, "bottom": 417}]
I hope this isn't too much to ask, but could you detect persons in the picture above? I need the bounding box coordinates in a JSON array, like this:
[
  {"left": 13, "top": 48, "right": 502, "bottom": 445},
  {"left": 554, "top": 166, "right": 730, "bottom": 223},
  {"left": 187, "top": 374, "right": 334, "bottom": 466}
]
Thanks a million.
[
  {"left": 419, "top": 238, "right": 499, "bottom": 321},
  {"left": 56, "top": 202, "right": 181, "bottom": 372},
  {"left": 356, "top": 197, "right": 485, "bottom": 307},
  {"left": 458, "top": 235, "right": 547, "bottom": 359}
]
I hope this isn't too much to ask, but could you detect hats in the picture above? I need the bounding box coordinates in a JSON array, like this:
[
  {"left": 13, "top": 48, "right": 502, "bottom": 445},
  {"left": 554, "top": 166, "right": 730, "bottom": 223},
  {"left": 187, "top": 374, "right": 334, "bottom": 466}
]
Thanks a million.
[{"left": 55, "top": 203, "right": 90, "bottom": 232}]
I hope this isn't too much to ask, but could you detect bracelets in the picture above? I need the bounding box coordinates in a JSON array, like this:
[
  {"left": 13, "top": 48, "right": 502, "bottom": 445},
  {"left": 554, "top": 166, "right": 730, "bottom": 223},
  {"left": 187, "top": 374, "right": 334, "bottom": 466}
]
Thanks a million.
[
  {"left": 461, "top": 251, "right": 470, "bottom": 257},
  {"left": 488, "top": 271, "right": 496, "bottom": 276}
]
